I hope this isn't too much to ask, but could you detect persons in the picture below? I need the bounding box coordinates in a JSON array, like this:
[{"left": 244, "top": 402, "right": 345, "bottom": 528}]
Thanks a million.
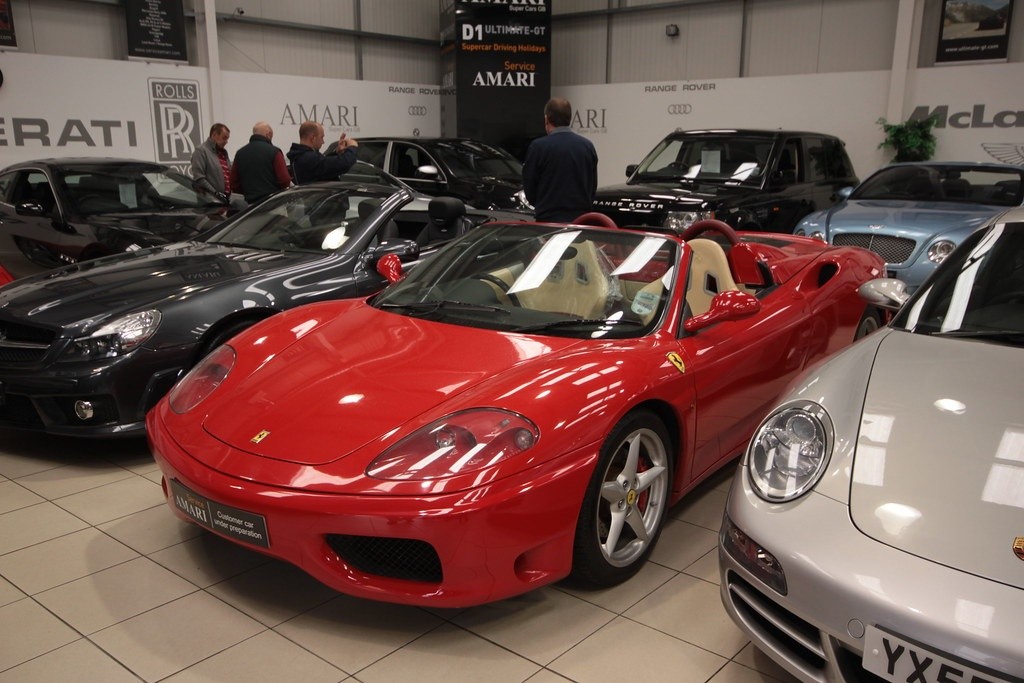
[
  {"left": 522, "top": 97, "right": 599, "bottom": 224},
  {"left": 286, "top": 121, "right": 359, "bottom": 227},
  {"left": 191, "top": 123, "right": 232, "bottom": 201},
  {"left": 229, "top": 121, "right": 290, "bottom": 206}
]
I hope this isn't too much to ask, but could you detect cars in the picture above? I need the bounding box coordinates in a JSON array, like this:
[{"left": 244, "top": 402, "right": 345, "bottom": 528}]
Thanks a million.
[
  {"left": 222, "top": 152, "right": 462, "bottom": 217},
  {"left": 978, "top": 15, "right": 1006, "bottom": 31},
  {"left": 0, "top": 153, "right": 310, "bottom": 286},
  {"left": 719, "top": 203, "right": 1023, "bottom": 683},
  {"left": 791, "top": 162, "right": 1024, "bottom": 316}
]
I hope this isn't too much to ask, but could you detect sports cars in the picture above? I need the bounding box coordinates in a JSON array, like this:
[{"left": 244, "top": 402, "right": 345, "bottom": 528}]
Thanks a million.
[
  {"left": 0, "top": 180, "right": 532, "bottom": 458},
  {"left": 146, "top": 206, "right": 892, "bottom": 611}
]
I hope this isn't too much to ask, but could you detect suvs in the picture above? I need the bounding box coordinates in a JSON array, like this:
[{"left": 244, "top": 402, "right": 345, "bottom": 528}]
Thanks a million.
[
  {"left": 324, "top": 134, "right": 531, "bottom": 208},
  {"left": 590, "top": 125, "right": 860, "bottom": 236}
]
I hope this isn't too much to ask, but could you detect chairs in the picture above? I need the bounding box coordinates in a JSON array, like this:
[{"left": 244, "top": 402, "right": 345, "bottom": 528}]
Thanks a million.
[
  {"left": 433, "top": 149, "right": 474, "bottom": 181},
  {"left": 910, "top": 168, "right": 945, "bottom": 202},
  {"left": 415, "top": 197, "right": 473, "bottom": 247},
  {"left": 985, "top": 179, "right": 1024, "bottom": 207},
  {"left": 630, "top": 238, "right": 743, "bottom": 334},
  {"left": 399, "top": 153, "right": 419, "bottom": 178},
  {"left": 347, "top": 198, "right": 399, "bottom": 247},
  {"left": 518, "top": 238, "right": 609, "bottom": 324},
  {"left": 696, "top": 140, "right": 732, "bottom": 174},
  {"left": 941, "top": 170, "right": 973, "bottom": 200}
]
